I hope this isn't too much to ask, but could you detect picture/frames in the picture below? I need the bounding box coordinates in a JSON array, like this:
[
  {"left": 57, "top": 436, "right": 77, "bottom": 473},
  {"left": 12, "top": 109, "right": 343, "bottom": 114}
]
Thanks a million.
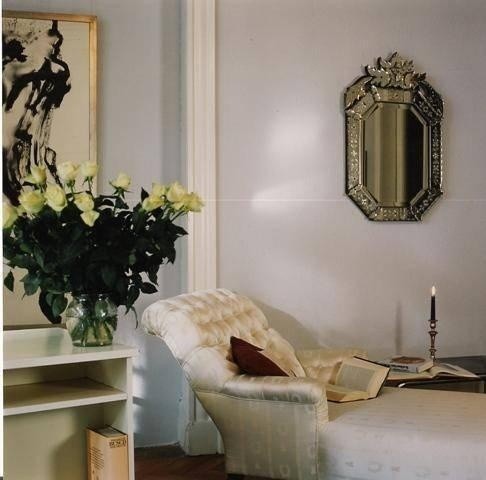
[{"left": 0, "top": 9, "right": 100, "bottom": 330}]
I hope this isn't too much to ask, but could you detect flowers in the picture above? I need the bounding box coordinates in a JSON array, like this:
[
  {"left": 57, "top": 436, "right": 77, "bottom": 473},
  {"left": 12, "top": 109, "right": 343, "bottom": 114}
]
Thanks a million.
[{"left": 2, "top": 159, "right": 205, "bottom": 349}]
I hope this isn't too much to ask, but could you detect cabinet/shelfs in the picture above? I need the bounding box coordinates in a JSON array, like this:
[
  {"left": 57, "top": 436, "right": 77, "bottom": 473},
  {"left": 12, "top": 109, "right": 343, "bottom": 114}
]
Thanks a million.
[{"left": 3, "top": 326, "right": 135, "bottom": 480}]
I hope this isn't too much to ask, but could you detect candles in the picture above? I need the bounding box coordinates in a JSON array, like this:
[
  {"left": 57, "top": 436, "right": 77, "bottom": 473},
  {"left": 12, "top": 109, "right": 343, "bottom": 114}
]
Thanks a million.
[{"left": 430, "top": 294, "right": 438, "bottom": 323}]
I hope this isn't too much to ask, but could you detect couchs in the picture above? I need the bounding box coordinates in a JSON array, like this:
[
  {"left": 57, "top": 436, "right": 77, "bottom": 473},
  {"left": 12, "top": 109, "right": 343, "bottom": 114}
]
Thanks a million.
[{"left": 140, "top": 285, "right": 486, "bottom": 480}]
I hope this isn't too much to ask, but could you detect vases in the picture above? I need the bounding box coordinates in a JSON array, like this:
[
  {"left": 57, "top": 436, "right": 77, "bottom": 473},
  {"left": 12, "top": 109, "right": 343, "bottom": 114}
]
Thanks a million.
[{"left": 63, "top": 290, "right": 119, "bottom": 349}]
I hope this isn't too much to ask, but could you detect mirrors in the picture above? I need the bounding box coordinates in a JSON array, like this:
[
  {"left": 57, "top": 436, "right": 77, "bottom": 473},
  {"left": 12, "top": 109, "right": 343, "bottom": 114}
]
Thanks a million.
[{"left": 343, "top": 51, "right": 443, "bottom": 222}]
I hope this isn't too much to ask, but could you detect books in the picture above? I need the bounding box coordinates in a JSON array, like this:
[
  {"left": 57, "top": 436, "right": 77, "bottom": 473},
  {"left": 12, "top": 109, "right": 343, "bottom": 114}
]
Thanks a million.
[
  {"left": 85, "top": 423, "right": 129, "bottom": 480},
  {"left": 375, "top": 353, "right": 434, "bottom": 373},
  {"left": 324, "top": 356, "right": 391, "bottom": 403},
  {"left": 385, "top": 361, "right": 479, "bottom": 382}
]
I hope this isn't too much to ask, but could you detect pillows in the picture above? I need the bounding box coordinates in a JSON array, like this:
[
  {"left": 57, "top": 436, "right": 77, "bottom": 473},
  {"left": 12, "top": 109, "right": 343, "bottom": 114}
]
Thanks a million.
[{"left": 233, "top": 338, "right": 287, "bottom": 381}]
